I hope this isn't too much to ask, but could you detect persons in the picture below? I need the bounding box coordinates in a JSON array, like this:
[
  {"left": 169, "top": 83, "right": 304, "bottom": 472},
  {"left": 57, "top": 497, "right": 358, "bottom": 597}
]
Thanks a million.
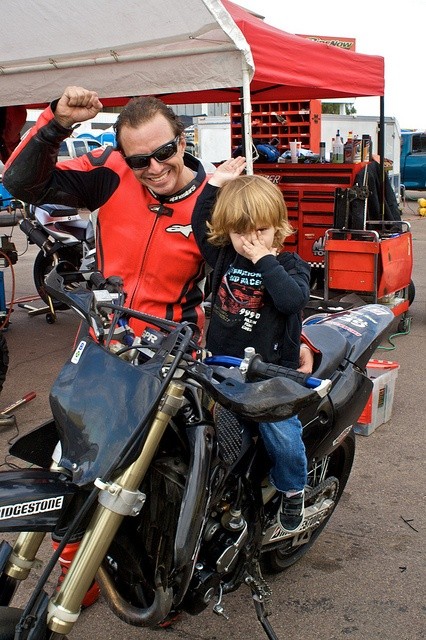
[
  {"left": 3, "top": 85, "right": 314, "bottom": 375},
  {"left": 191, "top": 156, "right": 311, "bottom": 534}
]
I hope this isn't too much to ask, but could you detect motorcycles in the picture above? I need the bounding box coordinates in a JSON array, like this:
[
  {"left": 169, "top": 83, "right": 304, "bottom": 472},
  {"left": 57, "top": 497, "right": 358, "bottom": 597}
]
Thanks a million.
[
  {"left": 17, "top": 203, "right": 87, "bottom": 311},
  {"left": 0, "top": 260, "right": 395, "bottom": 640}
]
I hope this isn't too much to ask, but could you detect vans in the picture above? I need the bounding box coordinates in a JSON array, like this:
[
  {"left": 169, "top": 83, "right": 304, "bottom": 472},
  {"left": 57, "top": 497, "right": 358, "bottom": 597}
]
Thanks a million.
[{"left": 56, "top": 137, "right": 101, "bottom": 162}]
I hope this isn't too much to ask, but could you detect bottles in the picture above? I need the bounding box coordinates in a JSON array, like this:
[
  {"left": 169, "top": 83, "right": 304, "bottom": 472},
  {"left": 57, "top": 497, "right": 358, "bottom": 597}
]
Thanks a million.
[
  {"left": 320, "top": 142, "right": 325, "bottom": 163},
  {"left": 354, "top": 139, "right": 362, "bottom": 163},
  {"left": 329, "top": 129, "right": 344, "bottom": 163},
  {"left": 345, "top": 132, "right": 353, "bottom": 163},
  {"left": 363, "top": 135, "right": 369, "bottom": 162}
]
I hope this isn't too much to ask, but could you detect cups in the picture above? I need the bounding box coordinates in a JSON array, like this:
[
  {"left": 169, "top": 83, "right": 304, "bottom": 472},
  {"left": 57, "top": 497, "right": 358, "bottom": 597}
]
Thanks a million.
[{"left": 289, "top": 141, "right": 302, "bottom": 164}]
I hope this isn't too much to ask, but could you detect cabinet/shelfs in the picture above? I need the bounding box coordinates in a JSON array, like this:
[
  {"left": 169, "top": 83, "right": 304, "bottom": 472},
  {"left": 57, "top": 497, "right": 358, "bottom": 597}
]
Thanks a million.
[
  {"left": 230, "top": 99, "right": 322, "bottom": 154},
  {"left": 211, "top": 163, "right": 370, "bottom": 267}
]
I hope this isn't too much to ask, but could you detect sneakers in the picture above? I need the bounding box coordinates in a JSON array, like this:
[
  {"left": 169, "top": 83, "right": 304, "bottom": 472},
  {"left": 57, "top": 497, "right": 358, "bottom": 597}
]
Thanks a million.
[{"left": 278, "top": 492, "right": 305, "bottom": 533}]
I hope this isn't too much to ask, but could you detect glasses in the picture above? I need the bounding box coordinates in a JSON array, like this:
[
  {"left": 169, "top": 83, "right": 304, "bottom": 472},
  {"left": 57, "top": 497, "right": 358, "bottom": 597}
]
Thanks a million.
[{"left": 123, "top": 134, "right": 179, "bottom": 170}]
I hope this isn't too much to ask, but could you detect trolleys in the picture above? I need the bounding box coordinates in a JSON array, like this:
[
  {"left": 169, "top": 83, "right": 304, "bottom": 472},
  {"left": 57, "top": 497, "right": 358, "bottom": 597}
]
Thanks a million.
[{"left": 323, "top": 220, "right": 413, "bottom": 334}]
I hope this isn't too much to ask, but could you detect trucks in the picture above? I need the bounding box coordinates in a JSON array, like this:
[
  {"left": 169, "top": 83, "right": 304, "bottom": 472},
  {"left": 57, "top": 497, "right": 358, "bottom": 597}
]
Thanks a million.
[
  {"left": 315, "top": 113, "right": 402, "bottom": 214},
  {"left": 400, "top": 129, "right": 426, "bottom": 191}
]
